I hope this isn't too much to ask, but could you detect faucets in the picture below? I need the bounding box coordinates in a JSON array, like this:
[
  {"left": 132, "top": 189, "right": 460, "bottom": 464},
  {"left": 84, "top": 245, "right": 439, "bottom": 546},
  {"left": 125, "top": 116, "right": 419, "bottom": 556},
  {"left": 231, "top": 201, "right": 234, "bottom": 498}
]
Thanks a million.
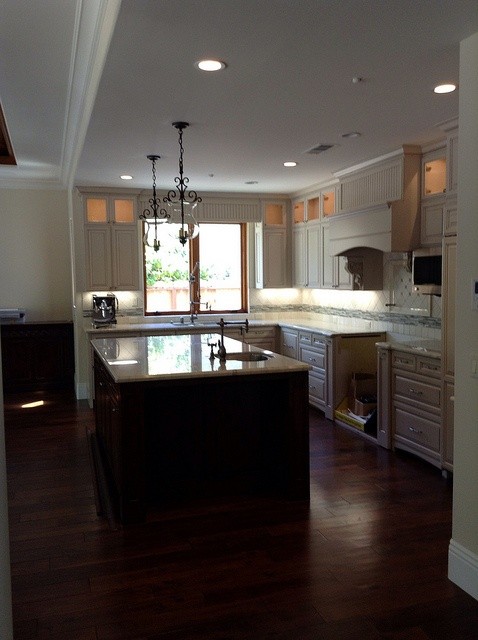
[
  {"left": 218, "top": 317, "right": 249, "bottom": 361},
  {"left": 189, "top": 301, "right": 208, "bottom": 323}
]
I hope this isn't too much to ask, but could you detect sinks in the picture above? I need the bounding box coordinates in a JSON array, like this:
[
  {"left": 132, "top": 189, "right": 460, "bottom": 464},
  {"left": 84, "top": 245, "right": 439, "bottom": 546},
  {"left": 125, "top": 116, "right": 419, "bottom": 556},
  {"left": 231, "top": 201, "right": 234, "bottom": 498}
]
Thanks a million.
[
  {"left": 214, "top": 353, "right": 272, "bottom": 362},
  {"left": 175, "top": 323, "right": 195, "bottom": 327},
  {"left": 202, "top": 321, "right": 225, "bottom": 324}
]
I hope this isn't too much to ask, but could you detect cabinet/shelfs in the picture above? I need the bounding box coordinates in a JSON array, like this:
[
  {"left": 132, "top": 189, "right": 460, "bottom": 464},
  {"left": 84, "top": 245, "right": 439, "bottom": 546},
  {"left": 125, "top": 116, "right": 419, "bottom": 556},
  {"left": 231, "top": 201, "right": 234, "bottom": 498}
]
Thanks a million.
[
  {"left": 445, "top": 130, "right": 457, "bottom": 200},
  {"left": 298, "top": 329, "right": 335, "bottom": 420},
  {"left": 263, "top": 227, "right": 291, "bottom": 290},
  {"left": 88, "top": 333, "right": 314, "bottom": 540},
  {"left": 419, "top": 197, "right": 444, "bottom": 247},
  {"left": 442, "top": 239, "right": 456, "bottom": 384},
  {"left": 445, "top": 380, "right": 453, "bottom": 474},
  {"left": 291, "top": 225, "right": 322, "bottom": 288},
  {"left": 85, "top": 225, "right": 140, "bottom": 291},
  {"left": 279, "top": 325, "right": 299, "bottom": 360},
  {"left": 322, "top": 221, "right": 352, "bottom": 290},
  {"left": 243, "top": 324, "right": 279, "bottom": 352},
  {"left": 388, "top": 349, "right": 445, "bottom": 469},
  {"left": 442, "top": 200, "right": 457, "bottom": 237}
]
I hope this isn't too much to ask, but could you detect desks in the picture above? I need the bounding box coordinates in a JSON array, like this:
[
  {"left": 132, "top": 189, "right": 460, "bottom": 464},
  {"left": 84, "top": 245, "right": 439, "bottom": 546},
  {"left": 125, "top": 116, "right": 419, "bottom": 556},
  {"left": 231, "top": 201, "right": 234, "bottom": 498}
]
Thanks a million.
[{"left": 1, "top": 316, "right": 74, "bottom": 398}]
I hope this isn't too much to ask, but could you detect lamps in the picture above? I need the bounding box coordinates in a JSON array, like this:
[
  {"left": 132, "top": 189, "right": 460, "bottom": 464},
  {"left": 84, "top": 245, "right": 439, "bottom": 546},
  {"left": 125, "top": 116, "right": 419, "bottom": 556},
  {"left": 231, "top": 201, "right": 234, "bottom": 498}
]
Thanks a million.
[
  {"left": 160, "top": 120, "right": 203, "bottom": 248},
  {"left": 138, "top": 154, "right": 170, "bottom": 254}
]
